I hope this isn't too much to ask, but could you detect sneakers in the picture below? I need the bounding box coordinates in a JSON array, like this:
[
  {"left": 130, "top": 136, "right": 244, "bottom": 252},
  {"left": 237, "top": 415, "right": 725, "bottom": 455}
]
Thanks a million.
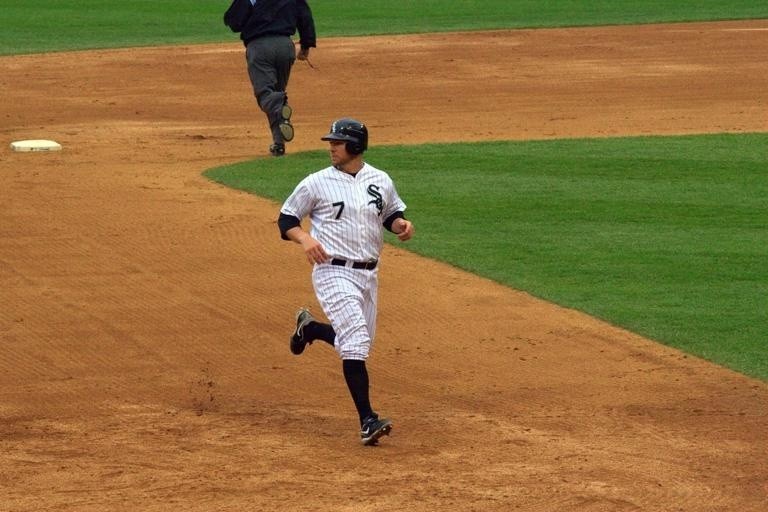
[
  {"left": 277, "top": 105, "right": 294, "bottom": 141},
  {"left": 290, "top": 309, "right": 314, "bottom": 355},
  {"left": 361, "top": 419, "right": 392, "bottom": 445}
]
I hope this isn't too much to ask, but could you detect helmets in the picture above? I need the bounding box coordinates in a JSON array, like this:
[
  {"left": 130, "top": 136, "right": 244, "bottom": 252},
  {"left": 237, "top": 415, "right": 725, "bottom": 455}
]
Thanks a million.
[
  {"left": 269, "top": 143, "right": 285, "bottom": 154},
  {"left": 320, "top": 118, "right": 368, "bottom": 154}
]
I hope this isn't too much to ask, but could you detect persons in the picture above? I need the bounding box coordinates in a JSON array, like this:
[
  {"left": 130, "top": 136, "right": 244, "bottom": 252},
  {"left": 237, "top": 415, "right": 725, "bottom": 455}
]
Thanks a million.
[
  {"left": 275, "top": 116, "right": 416, "bottom": 446},
  {"left": 223, "top": 0, "right": 318, "bottom": 156}
]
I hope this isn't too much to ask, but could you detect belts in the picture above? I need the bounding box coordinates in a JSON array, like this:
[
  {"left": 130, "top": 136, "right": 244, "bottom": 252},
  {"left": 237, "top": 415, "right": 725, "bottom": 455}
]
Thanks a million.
[{"left": 331, "top": 259, "right": 378, "bottom": 270}]
